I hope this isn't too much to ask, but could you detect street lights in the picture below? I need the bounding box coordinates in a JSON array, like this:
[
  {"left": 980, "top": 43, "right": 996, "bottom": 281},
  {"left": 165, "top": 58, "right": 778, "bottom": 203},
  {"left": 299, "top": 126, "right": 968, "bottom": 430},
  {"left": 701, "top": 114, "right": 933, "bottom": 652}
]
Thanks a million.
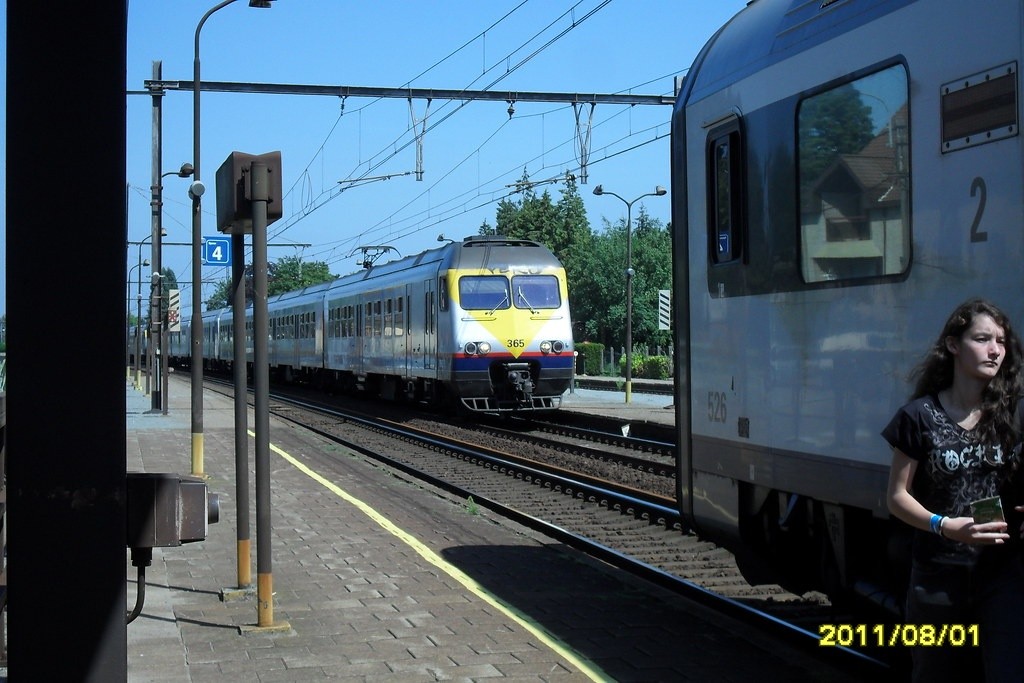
[
  {"left": 190, "top": 0, "right": 279, "bottom": 478},
  {"left": 136, "top": 228, "right": 167, "bottom": 387},
  {"left": 593, "top": 184, "right": 667, "bottom": 405},
  {"left": 149, "top": 163, "right": 194, "bottom": 410},
  {"left": 126, "top": 258, "right": 150, "bottom": 381}
]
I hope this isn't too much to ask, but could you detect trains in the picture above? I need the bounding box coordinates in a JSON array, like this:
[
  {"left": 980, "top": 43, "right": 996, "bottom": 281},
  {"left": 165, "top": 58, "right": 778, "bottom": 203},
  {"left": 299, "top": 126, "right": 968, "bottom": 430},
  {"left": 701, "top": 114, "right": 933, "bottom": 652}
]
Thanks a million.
[
  {"left": 670, "top": 0, "right": 1024, "bottom": 605},
  {"left": 126, "top": 234, "right": 579, "bottom": 427}
]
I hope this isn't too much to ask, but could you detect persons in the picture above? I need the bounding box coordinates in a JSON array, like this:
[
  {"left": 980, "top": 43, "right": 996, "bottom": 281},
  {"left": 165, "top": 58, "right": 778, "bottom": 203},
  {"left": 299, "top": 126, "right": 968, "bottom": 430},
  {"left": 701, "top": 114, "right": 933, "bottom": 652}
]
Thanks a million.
[{"left": 881, "top": 301, "right": 1024, "bottom": 683}]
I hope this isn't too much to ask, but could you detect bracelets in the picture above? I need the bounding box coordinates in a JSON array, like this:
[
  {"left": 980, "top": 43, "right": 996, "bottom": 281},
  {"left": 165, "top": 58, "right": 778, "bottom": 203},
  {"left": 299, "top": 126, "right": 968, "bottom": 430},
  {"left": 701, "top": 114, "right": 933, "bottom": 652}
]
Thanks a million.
[{"left": 930, "top": 515, "right": 949, "bottom": 537}]
